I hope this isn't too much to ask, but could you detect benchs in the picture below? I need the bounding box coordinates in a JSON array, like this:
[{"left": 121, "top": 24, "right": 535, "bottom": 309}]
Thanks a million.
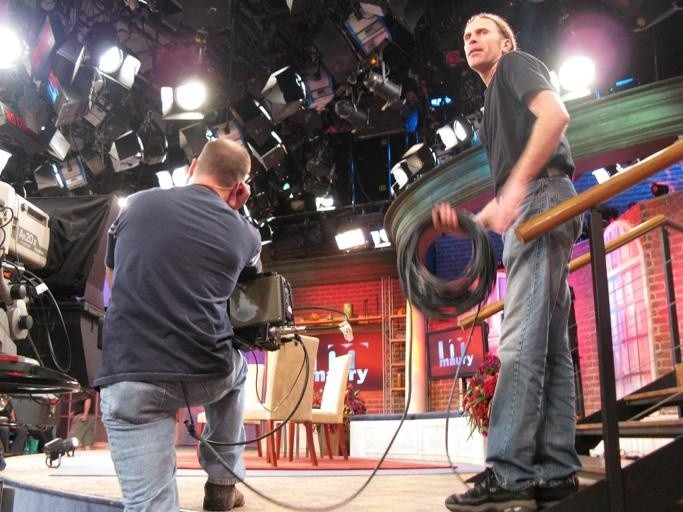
[{"left": 349, "top": 409, "right": 490, "bottom": 467}]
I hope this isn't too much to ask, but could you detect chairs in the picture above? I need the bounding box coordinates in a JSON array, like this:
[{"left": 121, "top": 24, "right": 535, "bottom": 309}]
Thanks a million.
[{"left": 196, "top": 334, "right": 353, "bottom": 466}]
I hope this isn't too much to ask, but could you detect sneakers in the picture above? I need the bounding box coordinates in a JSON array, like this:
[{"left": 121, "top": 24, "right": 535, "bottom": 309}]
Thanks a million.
[
  {"left": 443, "top": 466, "right": 579, "bottom": 512},
  {"left": 203, "top": 482, "right": 243, "bottom": 510}
]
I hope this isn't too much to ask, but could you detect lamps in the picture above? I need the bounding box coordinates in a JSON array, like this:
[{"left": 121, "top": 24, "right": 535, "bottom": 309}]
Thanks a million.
[
  {"left": 44, "top": 435, "right": 79, "bottom": 466},
  {"left": 0, "top": 1, "right": 475, "bottom": 254},
  {"left": 651, "top": 183, "right": 669, "bottom": 197}
]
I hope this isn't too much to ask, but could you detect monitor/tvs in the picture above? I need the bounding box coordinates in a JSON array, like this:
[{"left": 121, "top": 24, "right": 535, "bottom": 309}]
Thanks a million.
[{"left": 427, "top": 321, "right": 489, "bottom": 380}]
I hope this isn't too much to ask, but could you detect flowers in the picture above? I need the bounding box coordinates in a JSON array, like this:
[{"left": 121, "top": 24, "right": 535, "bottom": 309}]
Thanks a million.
[
  {"left": 310, "top": 382, "right": 367, "bottom": 431},
  {"left": 456, "top": 348, "right": 502, "bottom": 438}
]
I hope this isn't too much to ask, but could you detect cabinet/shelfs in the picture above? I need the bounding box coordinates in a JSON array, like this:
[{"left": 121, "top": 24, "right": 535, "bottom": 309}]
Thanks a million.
[{"left": 391, "top": 314, "right": 407, "bottom": 393}]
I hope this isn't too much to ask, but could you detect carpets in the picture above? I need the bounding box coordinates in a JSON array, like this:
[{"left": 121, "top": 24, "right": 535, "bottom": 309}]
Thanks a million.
[{"left": 173, "top": 454, "right": 458, "bottom": 470}]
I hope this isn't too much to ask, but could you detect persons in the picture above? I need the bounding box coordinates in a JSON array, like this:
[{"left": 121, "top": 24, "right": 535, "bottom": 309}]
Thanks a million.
[
  {"left": 0, "top": 392, "right": 28, "bottom": 455},
  {"left": 90, "top": 135, "right": 264, "bottom": 511},
  {"left": 428, "top": 9, "right": 583, "bottom": 512},
  {"left": 65, "top": 390, "right": 96, "bottom": 449}
]
